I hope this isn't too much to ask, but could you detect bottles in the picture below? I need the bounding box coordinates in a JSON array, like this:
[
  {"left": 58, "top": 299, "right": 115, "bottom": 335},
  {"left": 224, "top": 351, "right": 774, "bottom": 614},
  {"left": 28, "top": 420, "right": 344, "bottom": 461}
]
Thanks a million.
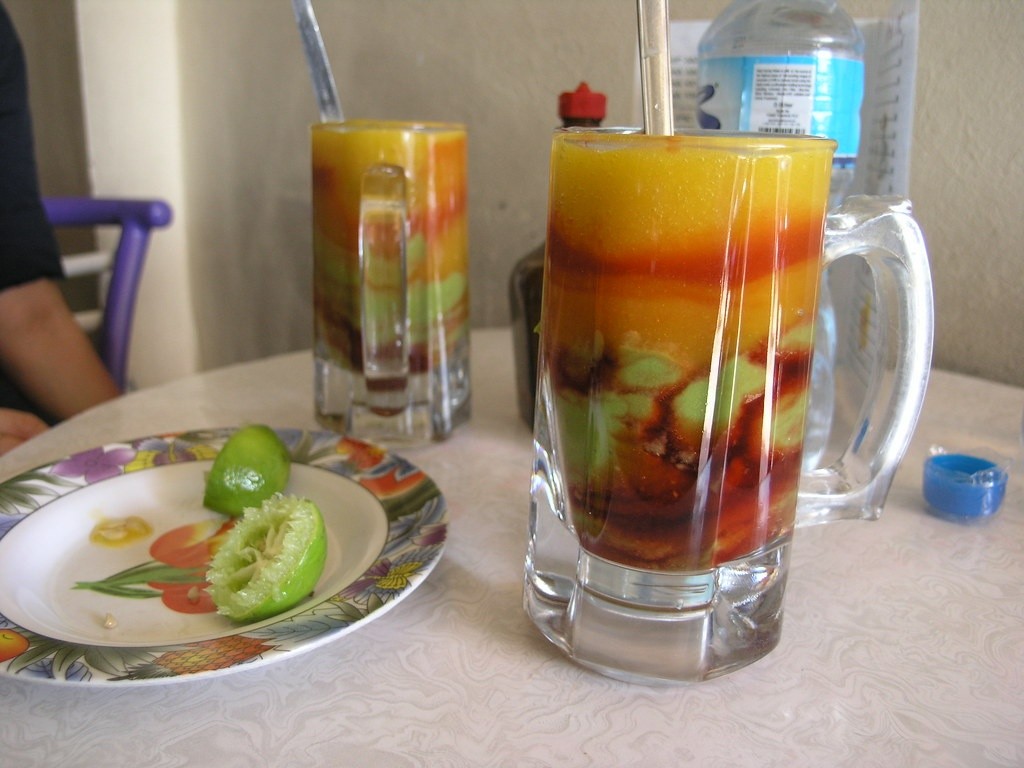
[
  {"left": 697, "top": 0, "right": 866, "bottom": 484},
  {"left": 511, "top": 81, "right": 608, "bottom": 427}
]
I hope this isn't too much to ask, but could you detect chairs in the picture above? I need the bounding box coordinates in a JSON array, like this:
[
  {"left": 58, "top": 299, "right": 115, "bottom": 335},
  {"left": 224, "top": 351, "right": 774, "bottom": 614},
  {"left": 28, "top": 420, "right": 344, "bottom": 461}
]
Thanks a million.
[{"left": 38, "top": 195, "right": 171, "bottom": 392}]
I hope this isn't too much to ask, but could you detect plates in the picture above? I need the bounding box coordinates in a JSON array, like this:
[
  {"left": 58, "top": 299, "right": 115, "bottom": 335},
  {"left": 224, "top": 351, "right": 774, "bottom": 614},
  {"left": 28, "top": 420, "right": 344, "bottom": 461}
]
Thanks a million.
[{"left": 0, "top": 422, "right": 446, "bottom": 688}]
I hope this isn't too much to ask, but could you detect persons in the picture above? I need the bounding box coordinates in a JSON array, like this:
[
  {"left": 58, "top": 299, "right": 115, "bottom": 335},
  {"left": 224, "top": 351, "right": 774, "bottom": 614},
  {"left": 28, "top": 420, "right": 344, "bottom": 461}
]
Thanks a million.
[{"left": 2, "top": 0, "right": 127, "bottom": 457}]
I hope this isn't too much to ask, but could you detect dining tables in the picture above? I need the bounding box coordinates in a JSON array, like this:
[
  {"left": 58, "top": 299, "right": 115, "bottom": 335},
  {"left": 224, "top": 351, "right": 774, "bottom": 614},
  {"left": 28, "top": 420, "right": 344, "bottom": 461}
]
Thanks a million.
[{"left": 2, "top": 326, "right": 1023, "bottom": 768}]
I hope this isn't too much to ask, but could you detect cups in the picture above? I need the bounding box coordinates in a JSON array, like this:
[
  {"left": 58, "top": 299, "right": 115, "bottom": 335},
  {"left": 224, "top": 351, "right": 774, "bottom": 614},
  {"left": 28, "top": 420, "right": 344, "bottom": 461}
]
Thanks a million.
[
  {"left": 526, "top": 116, "right": 836, "bottom": 683},
  {"left": 305, "top": 116, "right": 474, "bottom": 446},
  {"left": 641, "top": 4, "right": 938, "bottom": 523}
]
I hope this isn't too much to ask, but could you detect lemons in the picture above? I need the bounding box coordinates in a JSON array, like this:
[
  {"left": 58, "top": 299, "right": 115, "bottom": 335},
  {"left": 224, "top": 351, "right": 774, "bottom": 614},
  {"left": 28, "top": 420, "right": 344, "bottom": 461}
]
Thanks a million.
[{"left": 202, "top": 424, "right": 326, "bottom": 622}]
